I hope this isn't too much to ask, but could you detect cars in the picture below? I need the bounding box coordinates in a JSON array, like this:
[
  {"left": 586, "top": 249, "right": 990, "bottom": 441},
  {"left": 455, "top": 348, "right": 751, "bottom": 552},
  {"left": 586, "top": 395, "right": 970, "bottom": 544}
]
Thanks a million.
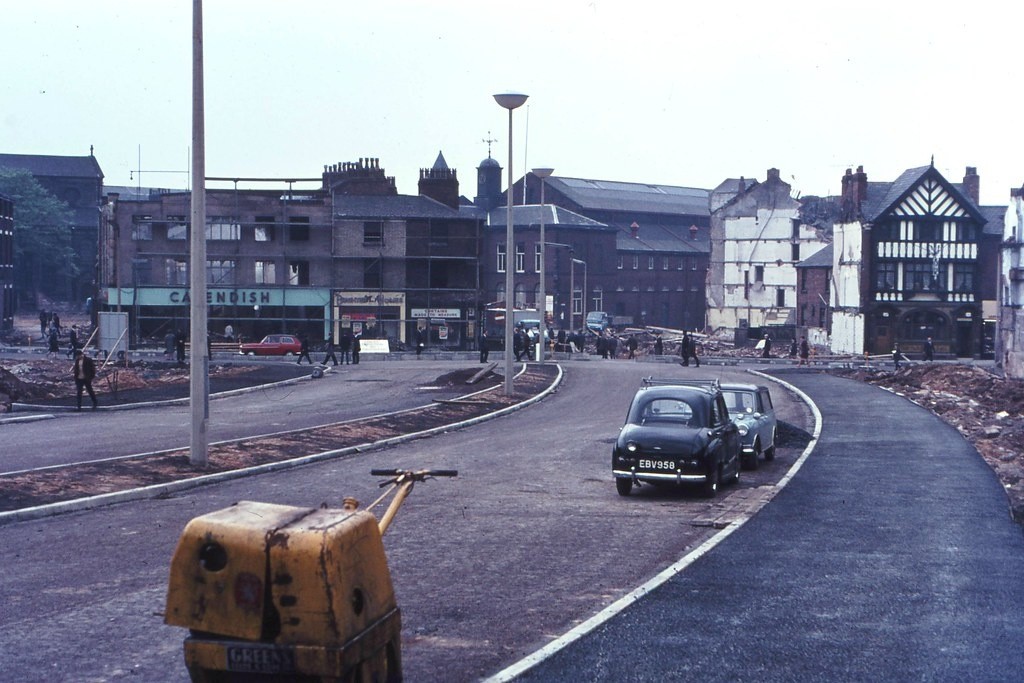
[
  {"left": 612, "top": 376, "right": 743, "bottom": 499},
  {"left": 240, "top": 334, "right": 302, "bottom": 357},
  {"left": 678, "top": 383, "right": 777, "bottom": 470}
]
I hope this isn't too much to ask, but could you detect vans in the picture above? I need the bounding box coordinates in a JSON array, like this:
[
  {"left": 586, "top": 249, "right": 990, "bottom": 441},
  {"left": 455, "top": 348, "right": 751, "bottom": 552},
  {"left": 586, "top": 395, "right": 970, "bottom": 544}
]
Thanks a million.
[{"left": 586, "top": 311, "right": 608, "bottom": 331}]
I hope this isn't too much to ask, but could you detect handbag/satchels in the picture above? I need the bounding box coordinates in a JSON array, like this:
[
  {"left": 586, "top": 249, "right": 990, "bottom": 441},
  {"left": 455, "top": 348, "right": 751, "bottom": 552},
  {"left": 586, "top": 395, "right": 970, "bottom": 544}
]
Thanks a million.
[
  {"left": 806, "top": 344, "right": 810, "bottom": 350},
  {"left": 420, "top": 343, "right": 424, "bottom": 346}
]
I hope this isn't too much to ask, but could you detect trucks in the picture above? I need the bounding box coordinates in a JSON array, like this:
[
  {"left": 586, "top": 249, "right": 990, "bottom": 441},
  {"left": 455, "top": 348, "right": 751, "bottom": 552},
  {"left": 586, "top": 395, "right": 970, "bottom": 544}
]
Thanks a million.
[{"left": 485, "top": 307, "right": 550, "bottom": 351}]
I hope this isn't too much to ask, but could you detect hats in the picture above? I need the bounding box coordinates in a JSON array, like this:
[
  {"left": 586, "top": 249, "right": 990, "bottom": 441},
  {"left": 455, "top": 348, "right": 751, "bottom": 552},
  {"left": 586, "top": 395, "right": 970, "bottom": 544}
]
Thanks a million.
[{"left": 764, "top": 334, "right": 769, "bottom": 336}]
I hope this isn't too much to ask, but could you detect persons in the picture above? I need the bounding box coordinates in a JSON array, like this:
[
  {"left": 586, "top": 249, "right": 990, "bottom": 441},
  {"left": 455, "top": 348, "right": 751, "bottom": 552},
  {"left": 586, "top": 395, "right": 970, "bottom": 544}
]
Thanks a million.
[
  {"left": 798, "top": 336, "right": 811, "bottom": 367},
  {"left": 352, "top": 332, "right": 361, "bottom": 364},
  {"left": 922, "top": 337, "right": 936, "bottom": 362},
  {"left": 416, "top": 327, "right": 424, "bottom": 355},
  {"left": 165, "top": 328, "right": 186, "bottom": 361},
  {"left": 224, "top": 324, "right": 234, "bottom": 343},
  {"left": 513, "top": 327, "right": 520, "bottom": 361},
  {"left": 892, "top": 343, "right": 901, "bottom": 369},
  {"left": 550, "top": 328, "right": 585, "bottom": 352},
  {"left": 339, "top": 331, "right": 351, "bottom": 365},
  {"left": 680, "top": 330, "right": 699, "bottom": 367},
  {"left": 320, "top": 332, "right": 338, "bottom": 366},
  {"left": 74, "top": 350, "right": 97, "bottom": 409},
  {"left": 625, "top": 333, "right": 637, "bottom": 359},
  {"left": 207, "top": 333, "right": 212, "bottom": 360},
  {"left": 598, "top": 333, "right": 617, "bottom": 359},
  {"left": 654, "top": 336, "right": 662, "bottom": 355},
  {"left": 297, "top": 334, "right": 313, "bottom": 365},
  {"left": 40, "top": 309, "right": 81, "bottom": 357},
  {"left": 517, "top": 327, "right": 534, "bottom": 360},
  {"left": 789, "top": 337, "right": 797, "bottom": 359},
  {"left": 762, "top": 333, "right": 771, "bottom": 358},
  {"left": 480, "top": 330, "right": 489, "bottom": 363}
]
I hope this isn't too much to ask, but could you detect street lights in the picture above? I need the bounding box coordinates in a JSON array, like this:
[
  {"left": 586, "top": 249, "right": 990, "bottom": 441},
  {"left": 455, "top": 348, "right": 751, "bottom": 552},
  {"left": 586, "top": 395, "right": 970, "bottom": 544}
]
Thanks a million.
[
  {"left": 492, "top": 93, "right": 529, "bottom": 396},
  {"left": 531, "top": 167, "right": 556, "bottom": 364}
]
